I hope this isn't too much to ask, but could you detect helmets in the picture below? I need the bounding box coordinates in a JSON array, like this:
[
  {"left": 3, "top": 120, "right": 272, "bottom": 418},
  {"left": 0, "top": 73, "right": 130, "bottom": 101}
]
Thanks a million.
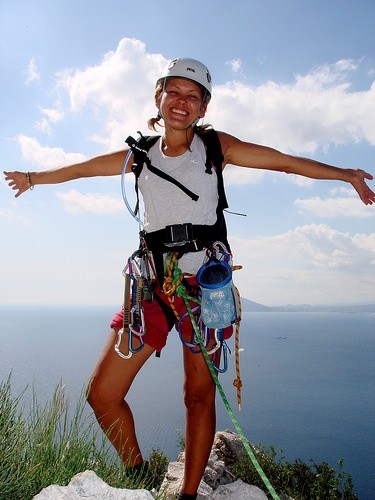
[{"left": 155, "top": 58, "right": 212, "bottom": 98}]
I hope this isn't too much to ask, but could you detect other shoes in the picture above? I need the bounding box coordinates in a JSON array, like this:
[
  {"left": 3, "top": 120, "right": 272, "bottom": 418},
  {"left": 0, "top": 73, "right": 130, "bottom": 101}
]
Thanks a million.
[
  {"left": 125, "top": 459, "right": 157, "bottom": 490},
  {"left": 176, "top": 490, "right": 197, "bottom": 500}
]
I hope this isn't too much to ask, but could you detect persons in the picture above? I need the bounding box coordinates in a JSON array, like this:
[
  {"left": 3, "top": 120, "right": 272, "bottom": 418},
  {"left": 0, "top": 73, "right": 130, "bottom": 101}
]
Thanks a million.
[{"left": 3, "top": 56, "right": 375, "bottom": 500}]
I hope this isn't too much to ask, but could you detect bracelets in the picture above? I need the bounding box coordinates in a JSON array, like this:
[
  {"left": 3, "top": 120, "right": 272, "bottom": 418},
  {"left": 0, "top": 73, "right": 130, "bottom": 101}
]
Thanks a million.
[{"left": 27, "top": 170, "right": 34, "bottom": 190}]
